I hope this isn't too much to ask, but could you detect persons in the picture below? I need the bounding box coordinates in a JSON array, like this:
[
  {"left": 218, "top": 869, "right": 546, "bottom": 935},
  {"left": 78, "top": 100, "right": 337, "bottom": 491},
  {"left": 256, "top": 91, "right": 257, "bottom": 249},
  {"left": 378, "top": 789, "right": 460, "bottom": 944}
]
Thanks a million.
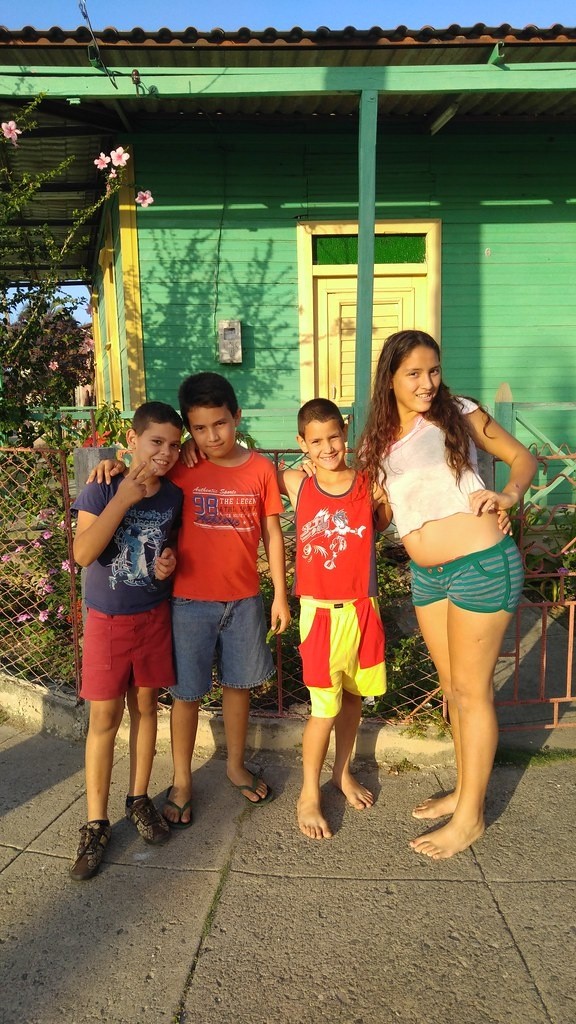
[
  {"left": 180, "top": 398, "right": 514, "bottom": 840},
  {"left": 297, "top": 330, "right": 539, "bottom": 860},
  {"left": 86, "top": 372, "right": 292, "bottom": 829},
  {"left": 69, "top": 401, "right": 184, "bottom": 882}
]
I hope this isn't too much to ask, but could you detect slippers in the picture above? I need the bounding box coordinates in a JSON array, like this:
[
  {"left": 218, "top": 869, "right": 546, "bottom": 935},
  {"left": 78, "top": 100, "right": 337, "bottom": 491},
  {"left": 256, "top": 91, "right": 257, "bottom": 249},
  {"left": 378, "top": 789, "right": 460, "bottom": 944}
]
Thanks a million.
[
  {"left": 226, "top": 768, "right": 273, "bottom": 807},
  {"left": 163, "top": 786, "right": 192, "bottom": 829}
]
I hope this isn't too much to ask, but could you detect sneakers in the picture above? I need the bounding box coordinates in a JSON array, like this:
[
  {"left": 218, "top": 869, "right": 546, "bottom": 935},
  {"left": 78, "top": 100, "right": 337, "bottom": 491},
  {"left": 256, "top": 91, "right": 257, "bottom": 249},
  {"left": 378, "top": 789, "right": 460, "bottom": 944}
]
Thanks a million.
[
  {"left": 125, "top": 797, "right": 170, "bottom": 845},
  {"left": 69, "top": 822, "right": 112, "bottom": 880}
]
